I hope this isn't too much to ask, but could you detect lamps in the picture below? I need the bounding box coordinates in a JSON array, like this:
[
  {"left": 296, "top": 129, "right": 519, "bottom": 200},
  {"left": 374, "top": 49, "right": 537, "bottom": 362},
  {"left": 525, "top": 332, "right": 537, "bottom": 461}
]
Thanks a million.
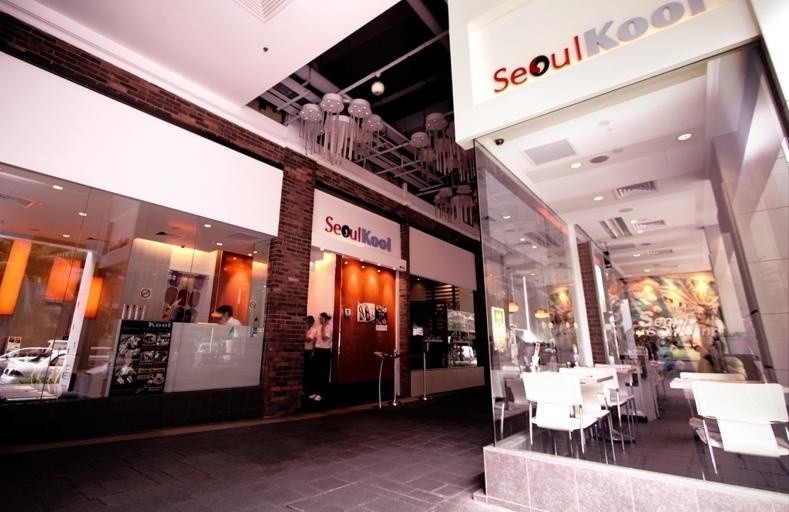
[
  {"left": 534, "top": 279, "right": 550, "bottom": 318},
  {"left": 507, "top": 272, "right": 519, "bottom": 312},
  {"left": 295, "top": 72, "right": 477, "bottom": 227}
]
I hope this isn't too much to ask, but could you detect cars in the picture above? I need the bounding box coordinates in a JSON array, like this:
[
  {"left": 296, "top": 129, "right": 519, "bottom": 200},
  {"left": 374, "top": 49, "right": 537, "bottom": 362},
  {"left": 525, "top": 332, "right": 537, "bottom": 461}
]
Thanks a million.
[{"left": 0, "top": 340, "right": 68, "bottom": 389}]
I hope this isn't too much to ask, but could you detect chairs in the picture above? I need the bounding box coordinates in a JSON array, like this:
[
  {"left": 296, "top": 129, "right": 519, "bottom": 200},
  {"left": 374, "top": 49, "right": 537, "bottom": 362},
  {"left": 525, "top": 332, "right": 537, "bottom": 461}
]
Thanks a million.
[{"left": 491, "top": 362, "right": 789, "bottom": 482}]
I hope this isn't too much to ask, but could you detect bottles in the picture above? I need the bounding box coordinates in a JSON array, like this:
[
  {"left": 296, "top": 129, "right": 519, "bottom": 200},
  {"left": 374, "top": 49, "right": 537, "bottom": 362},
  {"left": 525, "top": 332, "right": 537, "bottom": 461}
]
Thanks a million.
[{"left": 121, "top": 304, "right": 148, "bottom": 320}]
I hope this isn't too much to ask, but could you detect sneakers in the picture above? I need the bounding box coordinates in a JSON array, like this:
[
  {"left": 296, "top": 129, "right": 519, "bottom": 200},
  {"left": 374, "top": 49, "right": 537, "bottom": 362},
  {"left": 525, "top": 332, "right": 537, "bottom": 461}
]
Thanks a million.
[
  {"left": 313, "top": 395, "right": 321, "bottom": 401},
  {"left": 309, "top": 394, "right": 315, "bottom": 399}
]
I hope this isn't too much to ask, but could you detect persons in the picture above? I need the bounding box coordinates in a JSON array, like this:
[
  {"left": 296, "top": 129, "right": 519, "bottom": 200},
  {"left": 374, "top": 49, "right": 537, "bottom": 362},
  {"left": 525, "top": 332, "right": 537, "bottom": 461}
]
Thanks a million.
[
  {"left": 215, "top": 304, "right": 243, "bottom": 326},
  {"left": 183, "top": 307, "right": 199, "bottom": 323},
  {"left": 698, "top": 336, "right": 748, "bottom": 380},
  {"left": 301, "top": 315, "right": 317, "bottom": 397},
  {"left": 308, "top": 312, "right": 335, "bottom": 402},
  {"left": 358, "top": 305, "right": 365, "bottom": 320},
  {"left": 619, "top": 275, "right": 729, "bottom": 372},
  {"left": 365, "top": 305, "right": 371, "bottom": 322}
]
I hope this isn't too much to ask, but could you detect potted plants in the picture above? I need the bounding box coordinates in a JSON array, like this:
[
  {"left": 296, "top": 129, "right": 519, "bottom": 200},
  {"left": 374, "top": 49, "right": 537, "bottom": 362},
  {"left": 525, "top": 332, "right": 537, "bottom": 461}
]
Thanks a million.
[{"left": 18, "top": 371, "right": 60, "bottom": 394}]
